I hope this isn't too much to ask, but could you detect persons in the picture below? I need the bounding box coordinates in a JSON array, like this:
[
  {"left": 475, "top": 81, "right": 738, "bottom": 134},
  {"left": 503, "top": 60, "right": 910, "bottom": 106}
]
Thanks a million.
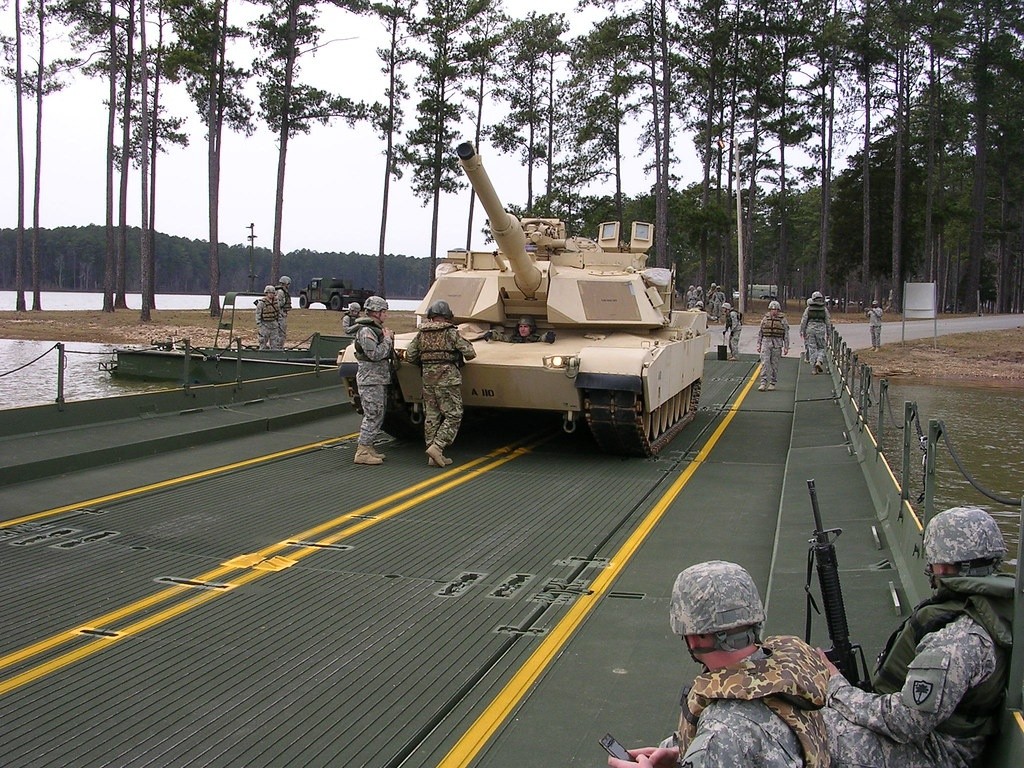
[
  {"left": 799, "top": 290, "right": 831, "bottom": 375},
  {"left": 256, "top": 285, "right": 280, "bottom": 350},
  {"left": 354, "top": 296, "right": 395, "bottom": 465},
  {"left": 818, "top": 506, "right": 1020, "bottom": 768},
  {"left": 756, "top": 300, "right": 790, "bottom": 390},
  {"left": 405, "top": 299, "right": 477, "bottom": 468},
  {"left": 866, "top": 301, "right": 884, "bottom": 352},
  {"left": 276, "top": 275, "right": 292, "bottom": 349},
  {"left": 342, "top": 302, "right": 362, "bottom": 336},
  {"left": 686, "top": 283, "right": 726, "bottom": 324},
  {"left": 721, "top": 303, "right": 742, "bottom": 361},
  {"left": 483, "top": 316, "right": 556, "bottom": 345},
  {"left": 608, "top": 560, "right": 830, "bottom": 768}
]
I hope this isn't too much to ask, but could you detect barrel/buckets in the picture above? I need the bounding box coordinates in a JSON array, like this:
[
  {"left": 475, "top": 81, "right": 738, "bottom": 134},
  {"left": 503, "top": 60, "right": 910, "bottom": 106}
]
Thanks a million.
[{"left": 718, "top": 345, "right": 727, "bottom": 360}]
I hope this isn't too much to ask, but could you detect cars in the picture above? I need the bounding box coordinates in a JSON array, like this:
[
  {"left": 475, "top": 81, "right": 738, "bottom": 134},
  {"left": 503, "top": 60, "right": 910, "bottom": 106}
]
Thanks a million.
[
  {"left": 825, "top": 295, "right": 878, "bottom": 306},
  {"left": 733, "top": 291, "right": 740, "bottom": 299},
  {"left": 945, "top": 303, "right": 952, "bottom": 311},
  {"left": 759, "top": 294, "right": 778, "bottom": 301}
]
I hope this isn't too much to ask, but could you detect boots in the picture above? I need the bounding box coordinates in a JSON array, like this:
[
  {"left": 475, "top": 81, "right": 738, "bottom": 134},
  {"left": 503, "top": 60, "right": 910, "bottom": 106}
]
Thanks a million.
[
  {"left": 816, "top": 362, "right": 824, "bottom": 373},
  {"left": 812, "top": 368, "right": 818, "bottom": 375},
  {"left": 369, "top": 445, "right": 385, "bottom": 459},
  {"left": 426, "top": 442, "right": 445, "bottom": 468},
  {"left": 353, "top": 445, "right": 383, "bottom": 464},
  {"left": 428, "top": 454, "right": 453, "bottom": 465}
]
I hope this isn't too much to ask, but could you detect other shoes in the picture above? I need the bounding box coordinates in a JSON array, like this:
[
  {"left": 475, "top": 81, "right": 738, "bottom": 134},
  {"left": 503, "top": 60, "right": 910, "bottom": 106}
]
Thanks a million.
[
  {"left": 875, "top": 347, "right": 881, "bottom": 353},
  {"left": 730, "top": 357, "right": 739, "bottom": 361},
  {"left": 869, "top": 346, "right": 875, "bottom": 351}
]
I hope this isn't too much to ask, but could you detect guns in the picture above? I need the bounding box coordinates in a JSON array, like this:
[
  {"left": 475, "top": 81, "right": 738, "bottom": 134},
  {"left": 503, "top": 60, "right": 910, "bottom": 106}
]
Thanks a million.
[{"left": 804, "top": 478, "right": 872, "bottom": 694}]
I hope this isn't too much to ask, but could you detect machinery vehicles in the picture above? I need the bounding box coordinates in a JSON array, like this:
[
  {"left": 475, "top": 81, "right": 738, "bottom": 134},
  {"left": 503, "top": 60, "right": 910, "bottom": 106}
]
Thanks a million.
[{"left": 335, "top": 141, "right": 712, "bottom": 459}]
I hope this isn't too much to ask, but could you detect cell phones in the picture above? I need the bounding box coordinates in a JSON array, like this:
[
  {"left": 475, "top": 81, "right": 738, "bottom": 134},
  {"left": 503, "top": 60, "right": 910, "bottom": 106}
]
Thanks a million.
[{"left": 598, "top": 732, "right": 637, "bottom": 761}]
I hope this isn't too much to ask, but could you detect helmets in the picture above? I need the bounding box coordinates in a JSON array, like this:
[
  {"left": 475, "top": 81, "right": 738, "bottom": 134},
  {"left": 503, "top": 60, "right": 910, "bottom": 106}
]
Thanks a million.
[
  {"left": 696, "top": 301, "right": 704, "bottom": 308},
  {"left": 768, "top": 301, "right": 781, "bottom": 310},
  {"left": 697, "top": 286, "right": 702, "bottom": 291},
  {"left": 689, "top": 285, "right": 694, "bottom": 290},
  {"left": 279, "top": 276, "right": 291, "bottom": 285},
  {"left": 813, "top": 292, "right": 822, "bottom": 300},
  {"left": 923, "top": 505, "right": 1009, "bottom": 566},
  {"left": 669, "top": 560, "right": 764, "bottom": 636},
  {"left": 873, "top": 300, "right": 880, "bottom": 306},
  {"left": 721, "top": 303, "right": 731, "bottom": 310},
  {"left": 711, "top": 283, "right": 717, "bottom": 287},
  {"left": 363, "top": 296, "right": 388, "bottom": 311},
  {"left": 716, "top": 285, "right": 721, "bottom": 289},
  {"left": 264, "top": 285, "right": 276, "bottom": 295},
  {"left": 807, "top": 298, "right": 813, "bottom": 307},
  {"left": 518, "top": 316, "right": 535, "bottom": 326},
  {"left": 350, "top": 302, "right": 361, "bottom": 311},
  {"left": 428, "top": 300, "right": 451, "bottom": 320}
]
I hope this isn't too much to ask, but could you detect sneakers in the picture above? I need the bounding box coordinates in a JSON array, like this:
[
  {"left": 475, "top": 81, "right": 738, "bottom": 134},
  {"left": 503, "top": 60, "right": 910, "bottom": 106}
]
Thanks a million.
[
  {"left": 768, "top": 384, "right": 775, "bottom": 390},
  {"left": 758, "top": 385, "right": 767, "bottom": 391}
]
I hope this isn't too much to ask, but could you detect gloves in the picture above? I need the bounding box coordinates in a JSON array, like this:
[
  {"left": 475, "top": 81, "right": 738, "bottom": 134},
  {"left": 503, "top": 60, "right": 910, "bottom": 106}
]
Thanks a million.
[
  {"left": 545, "top": 331, "right": 556, "bottom": 343},
  {"left": 484, "top": 331, "right": 493, "bottom": 342}
]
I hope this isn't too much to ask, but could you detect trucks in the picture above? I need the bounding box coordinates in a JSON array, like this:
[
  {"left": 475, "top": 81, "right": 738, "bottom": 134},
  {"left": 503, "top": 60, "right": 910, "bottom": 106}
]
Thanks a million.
[{"left": 299, "top": 278, "right": 377, "bottom": 311}]
